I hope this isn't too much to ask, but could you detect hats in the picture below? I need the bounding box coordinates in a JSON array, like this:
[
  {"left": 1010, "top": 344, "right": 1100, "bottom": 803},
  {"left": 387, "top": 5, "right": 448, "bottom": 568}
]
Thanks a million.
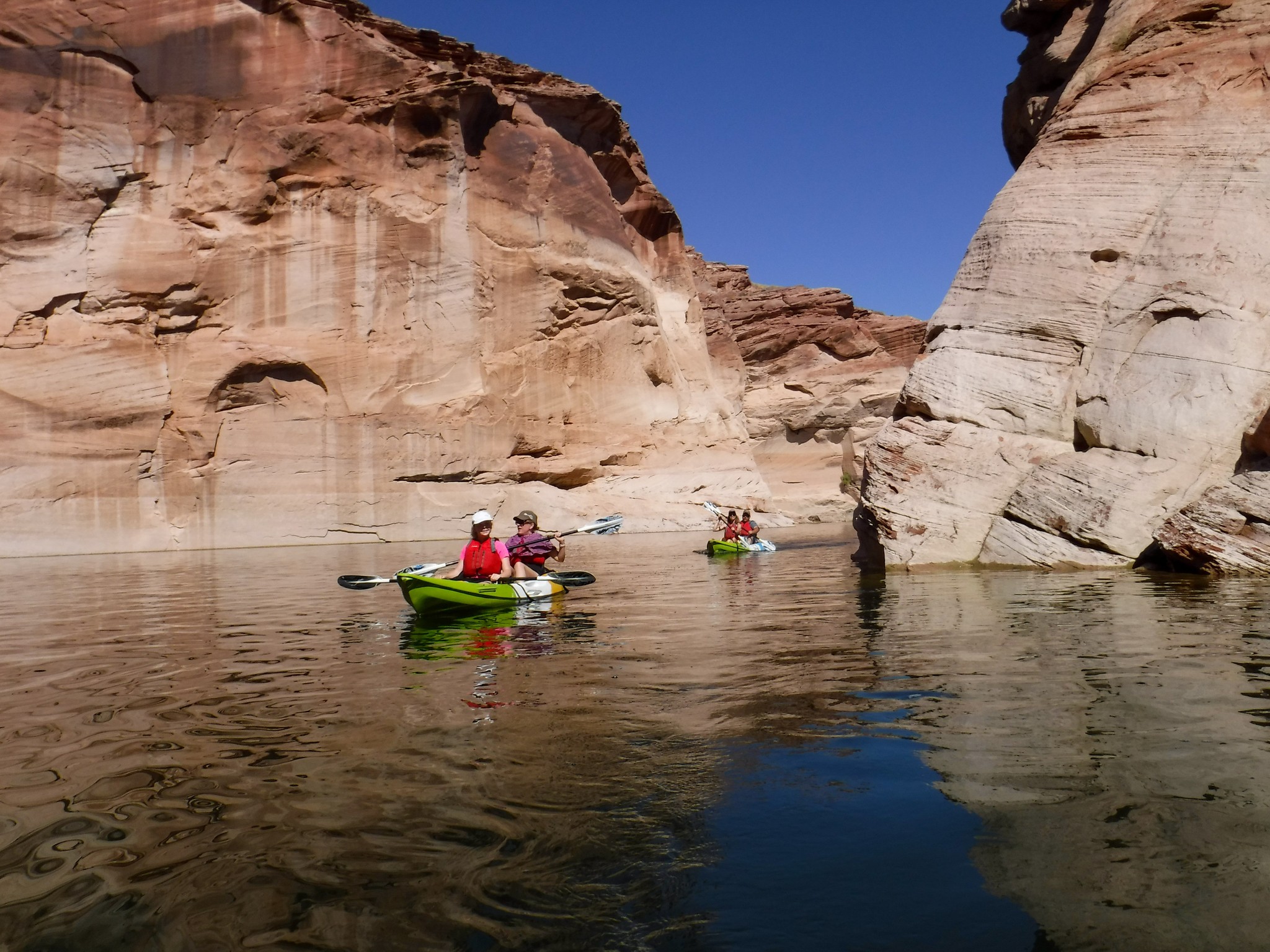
[
  {"left": 513, "top": 510, "right": 538, "bottom": 526},
  {"left": 743, "top": 510, "right": 750, "bottom": 516},
  {"left": 728, "top": 509, "right": 735, "bottom": 514},
  {"left": 472, "top": 511, "right": 493, "bottom": 527}
]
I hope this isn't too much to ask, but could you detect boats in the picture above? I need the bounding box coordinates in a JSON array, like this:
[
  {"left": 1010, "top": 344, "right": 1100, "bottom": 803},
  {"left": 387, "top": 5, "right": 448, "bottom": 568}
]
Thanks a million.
[
  {"left": 707, "top": 538, "right": 776, "bottom": 556},
  {"left": 396, "top": 568, "right": 570, "bottom": 618}
]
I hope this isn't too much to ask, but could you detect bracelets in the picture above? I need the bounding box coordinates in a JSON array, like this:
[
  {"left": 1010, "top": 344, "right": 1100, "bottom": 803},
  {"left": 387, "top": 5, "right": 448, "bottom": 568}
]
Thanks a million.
[{"left": 559, "top": 542, "right": 565, "bottom": 547}]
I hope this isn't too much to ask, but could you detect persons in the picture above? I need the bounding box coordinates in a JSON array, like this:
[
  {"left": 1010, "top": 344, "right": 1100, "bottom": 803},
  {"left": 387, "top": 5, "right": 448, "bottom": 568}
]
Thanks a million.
[
  {"left": 434, "top": 511, "right": 511, "bottom": 584},
  {"left": 712, "top": 509, "right": 760, "bottom": 544},
  {"left": 501, "top": 510, "right": 565, "bottom": 584}
]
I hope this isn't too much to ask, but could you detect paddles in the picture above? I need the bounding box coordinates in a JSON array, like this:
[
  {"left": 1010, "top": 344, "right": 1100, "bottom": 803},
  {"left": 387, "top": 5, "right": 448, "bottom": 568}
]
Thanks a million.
[
  {"left": 709, "top": 502, "right": 777, "bottom": 551},
  {"left": 338, "top": 571, "right": 598, "bottom": 591},
  {"left": 703, "top": 502, "right": 750, "bottom": 548},
  {"left": 392, "top": 515, "right": 627, "bottom": 578}
]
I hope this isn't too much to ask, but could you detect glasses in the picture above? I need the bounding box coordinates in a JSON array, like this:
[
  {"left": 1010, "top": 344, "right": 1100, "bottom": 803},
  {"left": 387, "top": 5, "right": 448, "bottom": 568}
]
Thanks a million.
[
  {"left": 515, "top": 520, "right": 532, "bottom": 526},
  {"left": 742, "top": 514, "right": 749, "bottom": 517},
  {"left": 729, "top": 513, "right": 736, "bottom": 516},
  {"left": 476, "top": 522, "right": 493, "bottom": 528}
]
